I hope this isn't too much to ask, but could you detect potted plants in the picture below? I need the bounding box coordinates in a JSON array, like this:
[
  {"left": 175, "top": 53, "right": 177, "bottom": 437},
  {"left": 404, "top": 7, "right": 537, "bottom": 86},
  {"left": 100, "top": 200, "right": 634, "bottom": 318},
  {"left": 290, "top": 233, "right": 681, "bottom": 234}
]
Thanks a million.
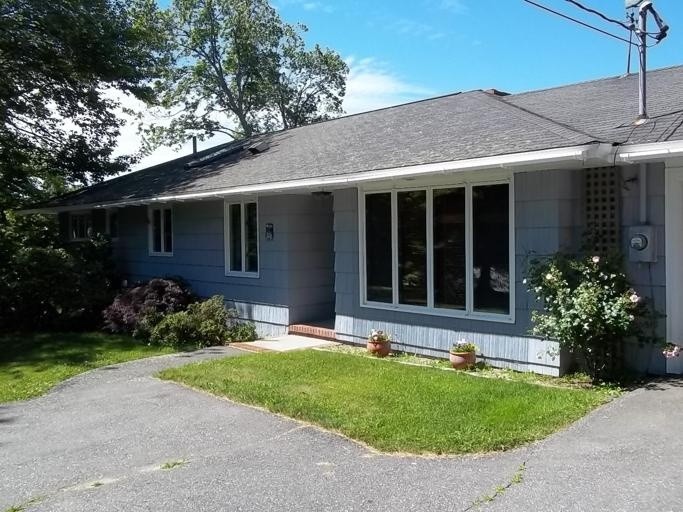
[
  {"left": 367, "top": 329, "right": 391, "bottom": 357},
  {"left": 449, "top": 338, "right": 479, "bottom": 369}
]
